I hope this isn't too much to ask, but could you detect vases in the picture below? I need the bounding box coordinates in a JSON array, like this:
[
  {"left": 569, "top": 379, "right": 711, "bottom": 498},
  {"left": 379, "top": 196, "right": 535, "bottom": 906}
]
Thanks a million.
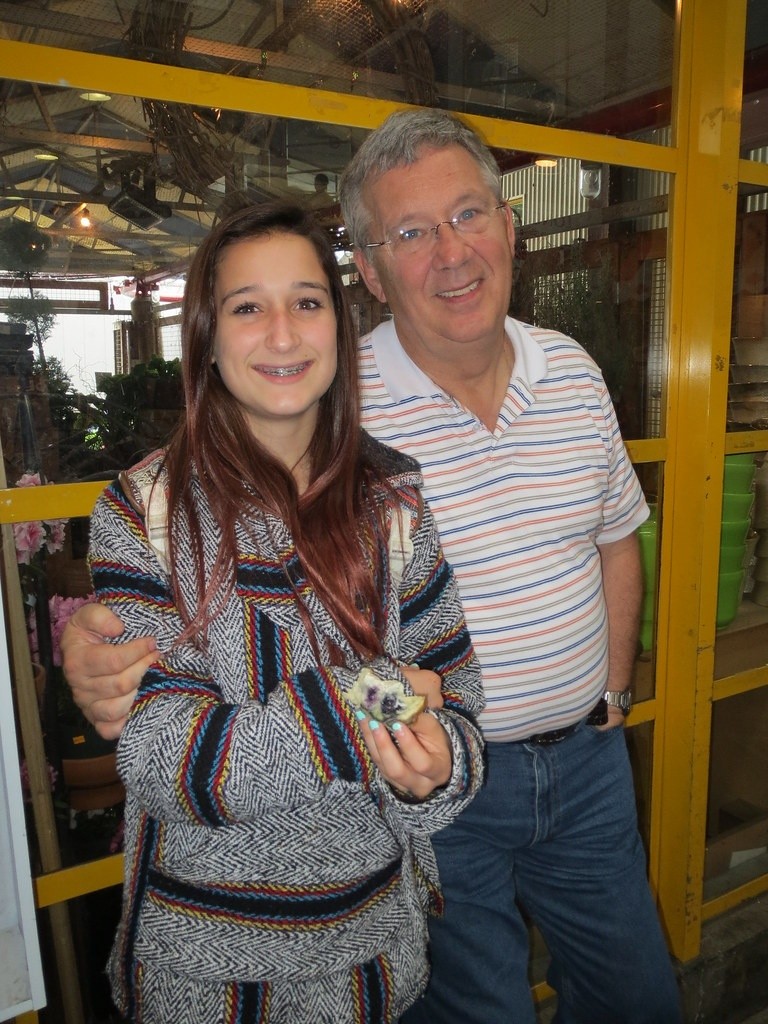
[
  {"left": 635, "top": 336, "right": 768, "bottom": 654},
  {"left": 10, "top": 662, "right": 47, "bottom": 724},
  {"left": 61, "top": 747, "right": 126, "bottom": 810}
]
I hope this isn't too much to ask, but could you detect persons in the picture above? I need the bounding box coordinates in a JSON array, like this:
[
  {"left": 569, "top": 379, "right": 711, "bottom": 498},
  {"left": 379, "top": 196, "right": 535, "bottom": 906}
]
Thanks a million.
[
  {"left": 60, "top": 107, "right": 686, "bottom": 1024},
  {"left": 87, "top": 200, "right": 489, "bottom": 1024}
]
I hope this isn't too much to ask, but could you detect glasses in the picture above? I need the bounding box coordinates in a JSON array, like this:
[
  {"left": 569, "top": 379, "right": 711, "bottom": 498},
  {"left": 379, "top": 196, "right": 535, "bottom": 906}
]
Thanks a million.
[{"left": 361, "top": 205, "right": 506, "bottom": 257}]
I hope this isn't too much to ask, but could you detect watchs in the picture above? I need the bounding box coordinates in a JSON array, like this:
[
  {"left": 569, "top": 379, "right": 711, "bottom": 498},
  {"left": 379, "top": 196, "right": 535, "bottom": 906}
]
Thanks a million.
[{"left": 602, "top": 688, "right": 632, "bottom": 716}]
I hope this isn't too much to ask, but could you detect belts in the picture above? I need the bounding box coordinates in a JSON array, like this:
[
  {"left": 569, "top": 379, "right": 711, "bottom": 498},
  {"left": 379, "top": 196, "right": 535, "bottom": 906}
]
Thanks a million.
[{"left": 505, "top": 698, "right": 608, "bottom": 746}]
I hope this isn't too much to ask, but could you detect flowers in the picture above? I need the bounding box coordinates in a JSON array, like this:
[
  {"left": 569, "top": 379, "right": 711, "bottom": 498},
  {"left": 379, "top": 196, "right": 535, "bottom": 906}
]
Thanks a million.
[
  {"left": 22, "top": 590, "right": 99, "bottom": 669},
  {"left": 5, "top": 467, "right": 67, "bottom": 568}
]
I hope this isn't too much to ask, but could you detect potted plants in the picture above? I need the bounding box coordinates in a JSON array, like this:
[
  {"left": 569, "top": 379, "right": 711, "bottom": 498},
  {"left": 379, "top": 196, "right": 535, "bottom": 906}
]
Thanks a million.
[{"left": 117, "top": 358, "right": 186, "bottom": 460}]
